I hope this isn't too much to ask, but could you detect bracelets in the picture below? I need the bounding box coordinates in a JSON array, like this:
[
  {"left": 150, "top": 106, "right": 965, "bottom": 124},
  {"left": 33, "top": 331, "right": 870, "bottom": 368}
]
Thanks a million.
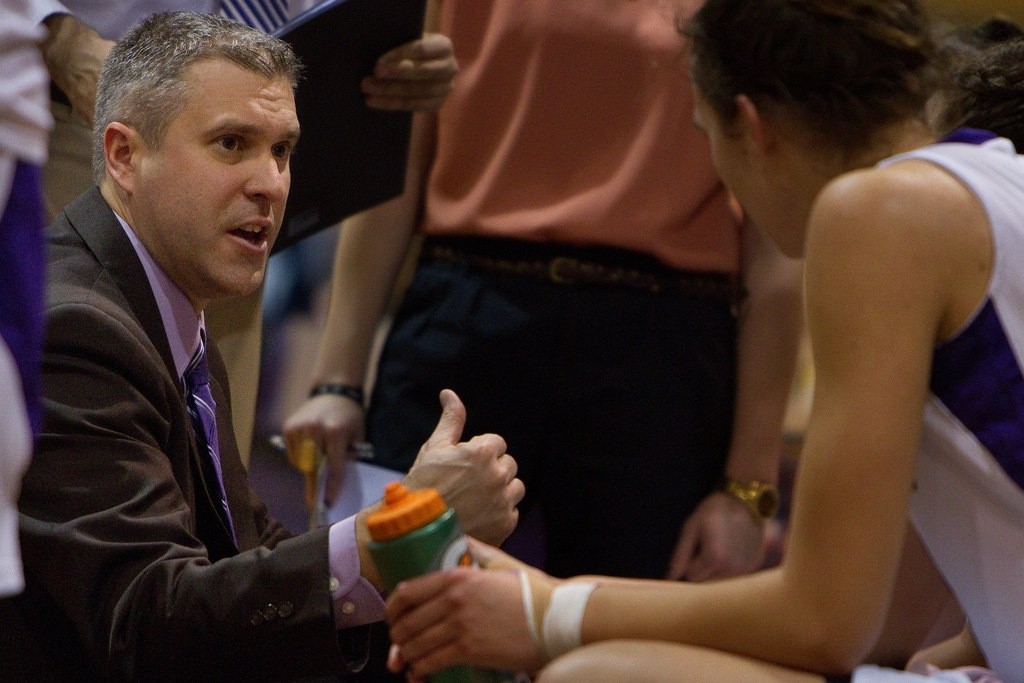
[
  {"left": 544, "top": 581, "right": 601, "bottom": 661},
  {"left": 308, "top": 384, "right": 364, "bottom": 407}
]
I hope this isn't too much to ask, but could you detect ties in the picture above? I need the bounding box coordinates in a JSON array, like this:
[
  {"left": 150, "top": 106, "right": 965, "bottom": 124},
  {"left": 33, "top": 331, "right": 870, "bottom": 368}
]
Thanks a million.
[{"left": 178, "top": 334, "right": 241, "bottom": 557}]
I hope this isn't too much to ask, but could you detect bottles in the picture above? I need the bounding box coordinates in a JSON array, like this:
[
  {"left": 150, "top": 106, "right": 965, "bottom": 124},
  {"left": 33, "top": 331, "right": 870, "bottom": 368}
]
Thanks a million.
[{"left": 369, "top": 483, "right": 513, "bottom": 683}]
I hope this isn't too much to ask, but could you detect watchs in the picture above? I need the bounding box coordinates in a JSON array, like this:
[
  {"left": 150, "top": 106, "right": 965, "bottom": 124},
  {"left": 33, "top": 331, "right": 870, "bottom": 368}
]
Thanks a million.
[{"left": 722, "top": 479, "right": 780, "bottom": 522}]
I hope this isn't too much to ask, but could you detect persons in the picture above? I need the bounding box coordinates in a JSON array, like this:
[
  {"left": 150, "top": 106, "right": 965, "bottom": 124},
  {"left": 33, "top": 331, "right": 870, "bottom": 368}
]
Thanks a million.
[
  {"left": 0, "top": 11, "right": 525, "bottom": 683},
  {"left": 0, "top": 0, "right": 423, "bottom": 596},
  {"left": 283, "top": 0, "right": 804, "bottom": 683},
  {"left": 382, "top": 1, "right": 1024, "bottom": 683},
  {"left": 751, "top": 2, "right": 1024, "bottom": 673}
]
